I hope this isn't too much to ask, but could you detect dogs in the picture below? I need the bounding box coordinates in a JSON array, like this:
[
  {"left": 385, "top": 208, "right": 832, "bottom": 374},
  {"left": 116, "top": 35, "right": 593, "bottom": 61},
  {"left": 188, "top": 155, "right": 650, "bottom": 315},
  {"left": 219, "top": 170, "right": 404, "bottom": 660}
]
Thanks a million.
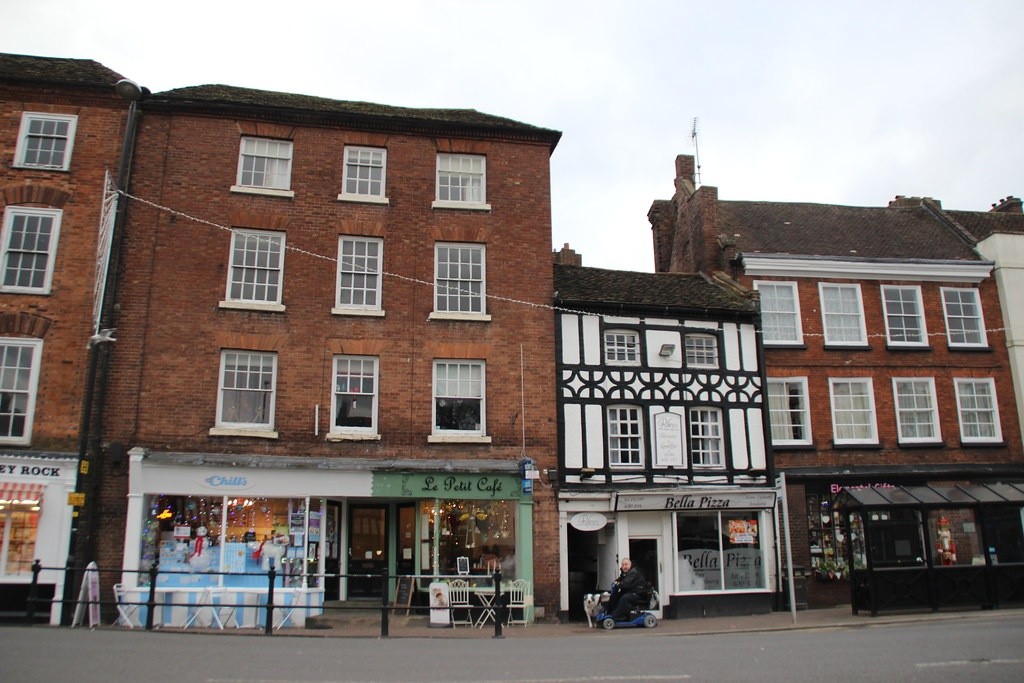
[{"left": 583, "top": 593, "right": 602, "bottom": 628}]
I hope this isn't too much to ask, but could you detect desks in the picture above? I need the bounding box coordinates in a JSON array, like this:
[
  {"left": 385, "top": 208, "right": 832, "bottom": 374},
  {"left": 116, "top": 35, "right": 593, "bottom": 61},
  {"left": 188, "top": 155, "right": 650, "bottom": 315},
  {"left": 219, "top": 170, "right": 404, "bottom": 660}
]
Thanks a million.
[
  {"left": 473, "top": 589, "right": 506, "bottom": 630},
  {"left": 128, "top": 584, "right": 285, "bottom": 631}
]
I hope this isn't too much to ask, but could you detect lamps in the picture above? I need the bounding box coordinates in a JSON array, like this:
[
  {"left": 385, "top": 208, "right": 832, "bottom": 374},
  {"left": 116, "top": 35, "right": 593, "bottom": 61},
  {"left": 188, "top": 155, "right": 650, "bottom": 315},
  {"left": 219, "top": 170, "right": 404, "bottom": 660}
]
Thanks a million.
[{"left": 581, "top": 467, "right": 596, "bottom": 481}]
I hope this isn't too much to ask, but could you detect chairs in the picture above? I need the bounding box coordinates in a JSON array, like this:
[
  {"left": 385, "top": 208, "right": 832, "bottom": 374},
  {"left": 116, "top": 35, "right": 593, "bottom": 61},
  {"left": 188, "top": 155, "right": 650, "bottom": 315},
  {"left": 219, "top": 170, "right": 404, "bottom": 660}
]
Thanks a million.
[
  {"left": 112, "top": 583, "right": 147, "bottom": 630},
  {"left": 271, "top": 588, "right": 303, "bottom": 633},
  {"left": 505, "top": 578, "right": 533, "bottom": 629},
  {"left": 207, "top": 584, "right": 243, "bottom": 631},
  {"left": 446, "top": 576, "right": 474, "bottom": 628}
]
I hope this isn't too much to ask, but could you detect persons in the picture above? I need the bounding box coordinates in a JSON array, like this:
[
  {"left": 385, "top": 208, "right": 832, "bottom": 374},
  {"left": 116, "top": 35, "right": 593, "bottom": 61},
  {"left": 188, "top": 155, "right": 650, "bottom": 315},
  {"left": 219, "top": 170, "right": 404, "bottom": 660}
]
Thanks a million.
[
  {"left": 456, "top": 541, "right": 516, "bottom": 570},
  {"left": 594, "top": 558, "right": 652, "bottom": 620}
]
